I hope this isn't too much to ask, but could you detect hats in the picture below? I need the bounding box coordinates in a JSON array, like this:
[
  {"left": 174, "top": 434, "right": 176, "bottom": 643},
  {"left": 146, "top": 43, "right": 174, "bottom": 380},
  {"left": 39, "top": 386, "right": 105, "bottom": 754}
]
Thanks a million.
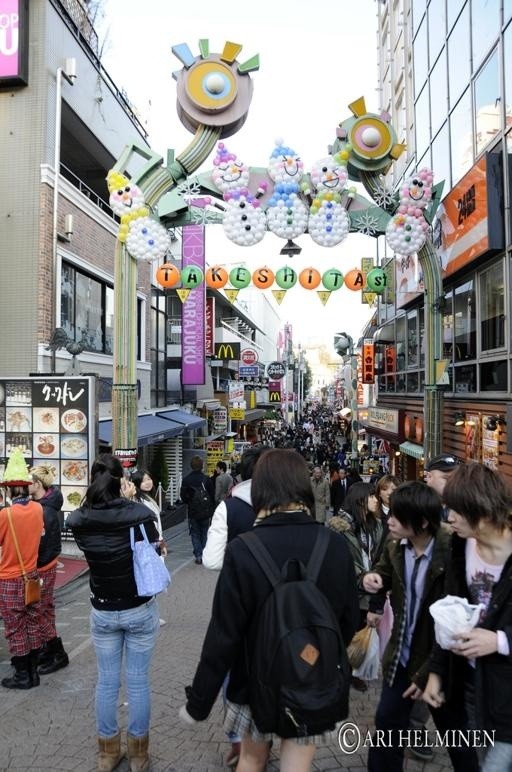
[
  {"left": 428, "top": 455, "right": 463, "bottom": 471},
  {"left": 0, "top": 446, "right": 33, "bottom": 486}
]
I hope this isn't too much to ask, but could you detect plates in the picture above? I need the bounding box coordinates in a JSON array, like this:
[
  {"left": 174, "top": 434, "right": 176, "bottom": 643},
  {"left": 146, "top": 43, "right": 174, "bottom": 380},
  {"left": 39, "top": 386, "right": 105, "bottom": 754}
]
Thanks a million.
[
  {"left": 61, "top": 437, "right": 88, "bottom": 457},
  {"left": 62, "top": 464, "right": 87, "bottom": 482},
  {"left": 37, "top": 408, "right": 59, "bottom": 433},
  {"left": 61, "top": 409, "right": 87, "bottom": 433},
  {"left": 36, "top": 443, "right": 57, "bottom": 456}
]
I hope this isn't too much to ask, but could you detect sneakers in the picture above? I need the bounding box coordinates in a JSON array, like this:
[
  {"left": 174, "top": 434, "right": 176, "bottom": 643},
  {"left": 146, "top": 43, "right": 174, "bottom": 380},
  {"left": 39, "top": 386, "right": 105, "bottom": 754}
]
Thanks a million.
[
  {"left": 403, "top": 726, "right": 432, "bottom": 758},
  {"left": 349, "top": 670, "right": 367, "bottom": 691},
  {"left": 226, "top": 741, "right": 240, "bottom": 766}
]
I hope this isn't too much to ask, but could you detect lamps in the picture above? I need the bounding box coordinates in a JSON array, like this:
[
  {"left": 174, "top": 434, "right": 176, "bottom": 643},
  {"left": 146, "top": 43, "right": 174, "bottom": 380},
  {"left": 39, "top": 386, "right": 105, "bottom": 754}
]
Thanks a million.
[
  {"left": 485, "top": 416, "right": 507, "bottom": 431},
  {"left": 454, "top": 412, "right": 465, "bottom": 426}
]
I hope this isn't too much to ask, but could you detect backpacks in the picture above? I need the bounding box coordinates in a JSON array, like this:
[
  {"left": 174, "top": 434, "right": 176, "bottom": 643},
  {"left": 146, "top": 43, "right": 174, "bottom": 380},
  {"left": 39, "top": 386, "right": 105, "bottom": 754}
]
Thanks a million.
[
  {"left": 235, "top": 523, "right": 349, "bottom": 739},
  {"left": 188, "top": 482, "right": 212, "bottom": 519}
]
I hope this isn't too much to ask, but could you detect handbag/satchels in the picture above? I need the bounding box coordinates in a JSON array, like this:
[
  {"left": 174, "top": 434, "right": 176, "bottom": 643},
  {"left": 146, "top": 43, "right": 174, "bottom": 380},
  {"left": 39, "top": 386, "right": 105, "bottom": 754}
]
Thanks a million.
[
  {"left": 23, "top": 578, "right": 40, "bottom": 605},
  {"left": 129, "top": 523, "right": 171, "bottom": 598}
]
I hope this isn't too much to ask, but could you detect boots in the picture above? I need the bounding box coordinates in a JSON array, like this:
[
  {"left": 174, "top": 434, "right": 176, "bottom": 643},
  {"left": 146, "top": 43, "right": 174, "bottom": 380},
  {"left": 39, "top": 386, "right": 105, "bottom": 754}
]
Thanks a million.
[
  {"left": 95, "top": 730, "right": 126, "bottom": 771},
  {"left": 126, "top": 733, "right": 149, "bottom": 772},
  {"left": 34, "top": 636, "right": 69, "bottom": 675},
  {"left": 2, "top": 647, "right": 39, "bottom": 689}
]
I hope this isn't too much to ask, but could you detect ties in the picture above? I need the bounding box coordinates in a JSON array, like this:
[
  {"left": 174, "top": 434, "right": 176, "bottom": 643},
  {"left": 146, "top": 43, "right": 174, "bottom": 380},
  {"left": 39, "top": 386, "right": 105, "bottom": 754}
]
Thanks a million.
[{"left": 342, "top": 480, "right": 344, "bottom": 488}]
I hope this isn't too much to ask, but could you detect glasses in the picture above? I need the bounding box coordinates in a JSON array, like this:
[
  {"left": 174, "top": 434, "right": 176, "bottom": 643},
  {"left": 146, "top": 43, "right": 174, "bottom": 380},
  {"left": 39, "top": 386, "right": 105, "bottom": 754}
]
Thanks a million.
[{"left": 429, "top": 457, "right": 461, "bottom": 467}]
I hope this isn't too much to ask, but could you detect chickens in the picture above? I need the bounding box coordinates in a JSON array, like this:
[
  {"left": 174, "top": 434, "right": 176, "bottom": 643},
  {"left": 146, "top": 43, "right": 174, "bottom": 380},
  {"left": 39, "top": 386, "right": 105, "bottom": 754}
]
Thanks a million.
[{"left": 43, "top": 324, "right": 91, "bottom": 357}]
